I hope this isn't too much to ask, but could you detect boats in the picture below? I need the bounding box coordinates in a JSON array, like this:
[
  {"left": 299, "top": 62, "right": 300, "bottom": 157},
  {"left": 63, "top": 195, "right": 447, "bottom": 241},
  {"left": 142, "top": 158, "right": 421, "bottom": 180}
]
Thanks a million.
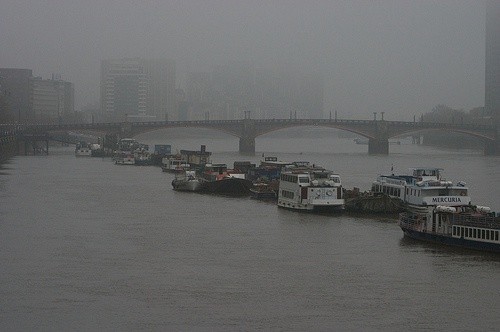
[
  {"left": 347, "top": 164, "right": 471, "bottom": 216},
  {"left": 76, "top": 137, "right": 291, "bottom": 199},
  {"left": 277, "top": 162, "right": 346, "bottom": 214},
  {"left": 399, "top": 202, "right": 500, "bottom": 251}
]
